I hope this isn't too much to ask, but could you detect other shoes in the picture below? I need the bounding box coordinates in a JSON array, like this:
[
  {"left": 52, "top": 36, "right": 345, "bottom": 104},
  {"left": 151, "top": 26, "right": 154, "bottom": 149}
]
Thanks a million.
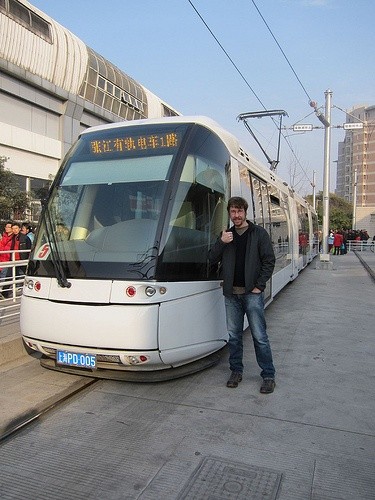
[{"left": 332, "top": 252, "right": 348, "bottom": 255}]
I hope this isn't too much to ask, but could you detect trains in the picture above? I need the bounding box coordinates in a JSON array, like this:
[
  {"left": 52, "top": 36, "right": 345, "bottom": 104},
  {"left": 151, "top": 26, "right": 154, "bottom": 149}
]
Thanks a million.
[{"left": 18, "top": 115, "right": 319, "bottom": 383}]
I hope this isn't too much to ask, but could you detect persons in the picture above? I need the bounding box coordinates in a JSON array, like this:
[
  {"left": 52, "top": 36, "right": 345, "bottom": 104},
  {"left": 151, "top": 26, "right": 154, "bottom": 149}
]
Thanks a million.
[
  {"left": 299, "top": 230, "right": 319, "bottom": 255},
  {"left": 209, "top": 196, "right": 276, "bottom": 393},
  {"left": 319, "top": 229, "right": 375, "bottom": 256},
  {"left": 0, "top": 222, "right": 39, "bottom": 300}
]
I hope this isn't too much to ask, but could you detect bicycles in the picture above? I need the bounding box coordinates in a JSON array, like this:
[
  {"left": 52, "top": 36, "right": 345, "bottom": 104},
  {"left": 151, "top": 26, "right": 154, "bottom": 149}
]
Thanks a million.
[{"left": 370, "top": 240, "right": 375, "bottom": 253}]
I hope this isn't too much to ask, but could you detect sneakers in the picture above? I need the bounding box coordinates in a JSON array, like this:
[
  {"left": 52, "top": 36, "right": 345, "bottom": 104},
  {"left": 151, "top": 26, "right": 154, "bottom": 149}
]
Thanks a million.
[
  {"left": 225, "top": 369, "right": 242, "bottom": 388},
  {"left": 260, "top": 376, "right": 276, "bottom": 393},
  {"left": 0, "top": 292, "right": 5, "bottom": 300}
]
